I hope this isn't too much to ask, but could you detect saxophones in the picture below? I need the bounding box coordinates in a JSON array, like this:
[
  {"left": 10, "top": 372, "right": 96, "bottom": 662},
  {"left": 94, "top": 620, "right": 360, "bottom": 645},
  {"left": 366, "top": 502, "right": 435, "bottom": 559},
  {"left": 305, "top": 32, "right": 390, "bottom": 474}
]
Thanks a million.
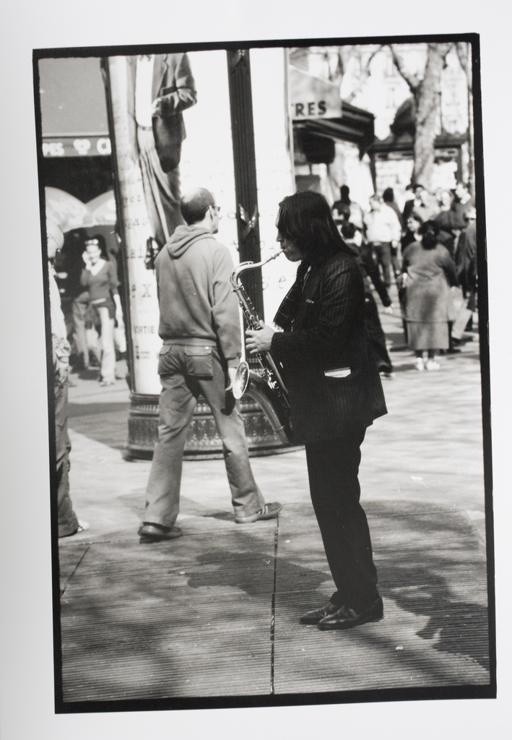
[{"left": 230, "top": 250, "right": 294, "bottom": 447}]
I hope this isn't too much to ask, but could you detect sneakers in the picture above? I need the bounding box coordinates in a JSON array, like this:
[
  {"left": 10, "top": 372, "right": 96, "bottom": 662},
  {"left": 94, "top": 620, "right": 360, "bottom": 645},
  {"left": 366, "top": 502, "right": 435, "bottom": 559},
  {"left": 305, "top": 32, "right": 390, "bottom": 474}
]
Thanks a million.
[
  {"left": 234, "top": 503, "right": 281, "bottom": 523},
  {"left": 138, "top": 523, "right": 182, "bottom": 538}
]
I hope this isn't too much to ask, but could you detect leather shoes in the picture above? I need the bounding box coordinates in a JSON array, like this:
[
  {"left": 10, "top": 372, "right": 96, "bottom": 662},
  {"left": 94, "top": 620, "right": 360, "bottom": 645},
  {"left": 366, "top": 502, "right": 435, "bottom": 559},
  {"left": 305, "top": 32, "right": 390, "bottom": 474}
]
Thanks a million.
[
  {"left": 318, "top": 597, "right": 382, "bottom": 630},
  {"left": 300, "top": 602, "right": 339, "bottom": 624}
]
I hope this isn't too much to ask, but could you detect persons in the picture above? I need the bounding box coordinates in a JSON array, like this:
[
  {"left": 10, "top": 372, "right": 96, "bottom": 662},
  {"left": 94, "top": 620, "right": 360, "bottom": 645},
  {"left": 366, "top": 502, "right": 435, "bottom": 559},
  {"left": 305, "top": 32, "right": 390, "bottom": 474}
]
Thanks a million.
[
  {"left": 80, "top": 239, "right": 126, "bottom": 387},
  {"left": 46, "top": 217, "right": 79, "bottom": 538},
  {"left": 245, "top": 191, "right": 388, "bottom": 630},
  {"left": 328, "top": 181, "right": 480, "bottom": 372},
  {"left": 125, "top": 52, "right": 197, "bottom": 250},
  {"left": 138, "top": 187, "right": 282, "bottom": 537}
]
